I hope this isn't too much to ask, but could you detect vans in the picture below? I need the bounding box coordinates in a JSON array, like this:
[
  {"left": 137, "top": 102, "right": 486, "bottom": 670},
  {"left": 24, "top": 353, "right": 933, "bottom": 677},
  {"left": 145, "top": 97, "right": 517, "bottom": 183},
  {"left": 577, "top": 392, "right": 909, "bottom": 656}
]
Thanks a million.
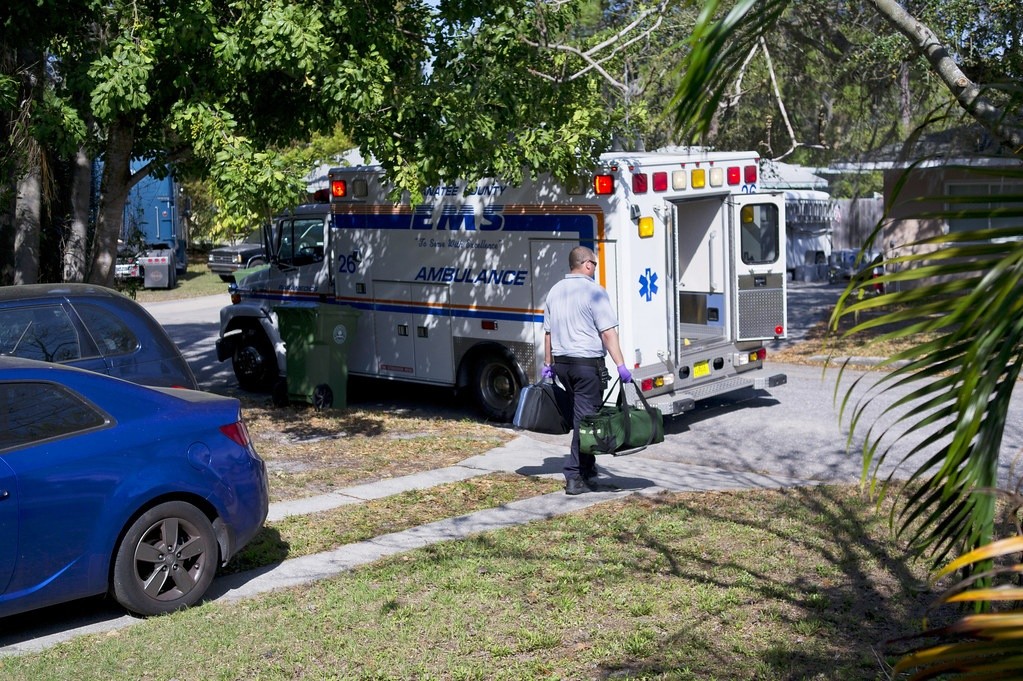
[{"left": 0, "top": 283, "right": 201, "bottom": 392}]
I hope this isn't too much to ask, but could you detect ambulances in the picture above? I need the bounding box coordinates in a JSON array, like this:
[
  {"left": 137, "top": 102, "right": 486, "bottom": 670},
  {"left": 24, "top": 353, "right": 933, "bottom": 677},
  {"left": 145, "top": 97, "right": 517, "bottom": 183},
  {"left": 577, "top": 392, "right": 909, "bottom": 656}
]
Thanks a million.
[{"left": 216, "top": 151, "right": 789, "bottom": 429}]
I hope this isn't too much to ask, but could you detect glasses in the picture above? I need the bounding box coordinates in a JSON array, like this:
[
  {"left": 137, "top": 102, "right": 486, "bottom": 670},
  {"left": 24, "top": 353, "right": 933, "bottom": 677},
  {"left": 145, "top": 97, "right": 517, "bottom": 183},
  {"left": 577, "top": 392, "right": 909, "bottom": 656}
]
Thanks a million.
[{"left": 581, "top": 259, "right": 598, "bottom": 268}]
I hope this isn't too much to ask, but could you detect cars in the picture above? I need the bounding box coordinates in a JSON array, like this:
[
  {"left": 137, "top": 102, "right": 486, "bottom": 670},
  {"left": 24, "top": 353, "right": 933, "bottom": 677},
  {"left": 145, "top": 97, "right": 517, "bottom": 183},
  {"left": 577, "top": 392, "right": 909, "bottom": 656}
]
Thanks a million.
[
  {"left": 207, "top": 227, "right": 268, "bottom": 282},
  {"left": 0, "top": 355, "right": 270, "bottom": 619}
]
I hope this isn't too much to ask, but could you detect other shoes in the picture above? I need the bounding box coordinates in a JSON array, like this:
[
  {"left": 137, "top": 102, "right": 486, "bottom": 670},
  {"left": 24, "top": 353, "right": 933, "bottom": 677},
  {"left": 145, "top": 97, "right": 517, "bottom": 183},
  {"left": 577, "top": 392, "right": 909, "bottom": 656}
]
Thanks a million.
[
  {"left": 580, "top": 465, "right": 598, "bottom": 478},
  {"left": 566, "top": 470, "right": 598, "bottom": 495}
]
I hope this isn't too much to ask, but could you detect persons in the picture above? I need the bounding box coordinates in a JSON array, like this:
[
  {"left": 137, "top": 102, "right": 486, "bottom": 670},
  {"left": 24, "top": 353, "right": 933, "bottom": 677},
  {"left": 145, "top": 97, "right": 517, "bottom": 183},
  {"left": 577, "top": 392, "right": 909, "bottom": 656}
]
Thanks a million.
[{"left": 540, "top": 245, "right": 631, "bottom": 494}]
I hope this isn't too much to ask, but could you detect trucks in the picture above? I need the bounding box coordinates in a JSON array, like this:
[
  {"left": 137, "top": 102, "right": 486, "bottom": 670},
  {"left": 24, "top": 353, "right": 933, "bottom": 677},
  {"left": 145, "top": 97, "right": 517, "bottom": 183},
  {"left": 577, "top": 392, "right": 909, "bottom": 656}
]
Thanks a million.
[{"left": 91, "top": 156, "right": 191, "bottom": 275}]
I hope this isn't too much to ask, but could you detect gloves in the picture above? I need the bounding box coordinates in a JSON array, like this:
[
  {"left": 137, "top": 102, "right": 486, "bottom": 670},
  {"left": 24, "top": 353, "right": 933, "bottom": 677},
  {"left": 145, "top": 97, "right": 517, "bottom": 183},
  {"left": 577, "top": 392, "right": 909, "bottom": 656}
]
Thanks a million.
[
  {"left": 542, "top": 365, "right": 555, "bottom": 379},
  {"left": 617, "top": 364, "right": 632, "bottom": 384}
]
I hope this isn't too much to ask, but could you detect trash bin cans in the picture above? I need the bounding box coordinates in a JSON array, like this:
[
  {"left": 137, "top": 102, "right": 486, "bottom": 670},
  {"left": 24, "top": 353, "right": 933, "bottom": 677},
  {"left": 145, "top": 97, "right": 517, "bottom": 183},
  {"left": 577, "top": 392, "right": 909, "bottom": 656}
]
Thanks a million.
[{"left": 273, "top": 301, "right": 363, "bottom": 410}]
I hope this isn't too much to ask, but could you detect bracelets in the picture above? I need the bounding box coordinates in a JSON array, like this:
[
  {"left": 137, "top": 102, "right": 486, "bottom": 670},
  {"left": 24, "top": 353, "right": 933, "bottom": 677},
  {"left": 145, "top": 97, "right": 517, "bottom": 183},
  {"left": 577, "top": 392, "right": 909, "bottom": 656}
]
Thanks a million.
[{"left": 544, "top": 363, "right": 552, "bottom": 366}]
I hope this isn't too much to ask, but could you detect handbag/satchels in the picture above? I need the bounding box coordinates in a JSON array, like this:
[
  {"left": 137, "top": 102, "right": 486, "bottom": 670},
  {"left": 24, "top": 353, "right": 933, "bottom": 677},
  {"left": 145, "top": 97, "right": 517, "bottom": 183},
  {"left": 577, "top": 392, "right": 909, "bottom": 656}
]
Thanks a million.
[
  {"left": 579, "top": 377, "right": 665, "bottom": 457},
  {"left": 512, "top": 370, "right": 571, "bottom": 434}
]
